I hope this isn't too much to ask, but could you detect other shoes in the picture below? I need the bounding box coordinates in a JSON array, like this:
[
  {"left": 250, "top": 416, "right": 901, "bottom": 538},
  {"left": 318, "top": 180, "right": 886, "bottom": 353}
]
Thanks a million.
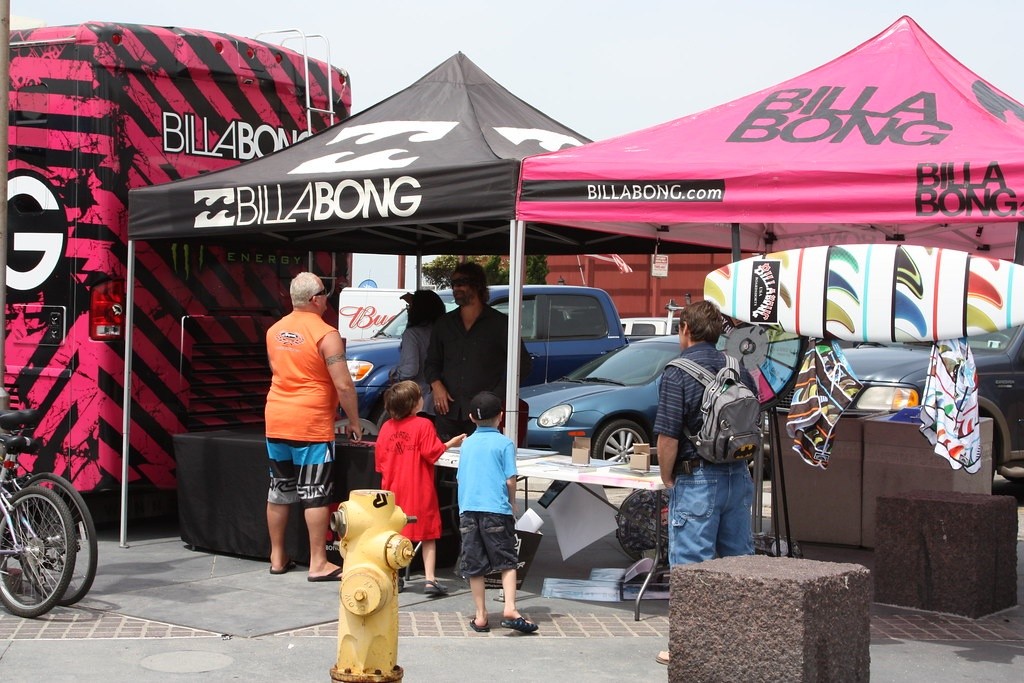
[{"left": 656, "top": 651, "right": 670, "bottom": 666}]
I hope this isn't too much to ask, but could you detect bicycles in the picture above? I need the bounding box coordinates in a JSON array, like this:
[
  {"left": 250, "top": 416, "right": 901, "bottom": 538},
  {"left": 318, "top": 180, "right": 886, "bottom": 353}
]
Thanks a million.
[{"left": 0, "top": 407, "right": 99, "bottom": 618}]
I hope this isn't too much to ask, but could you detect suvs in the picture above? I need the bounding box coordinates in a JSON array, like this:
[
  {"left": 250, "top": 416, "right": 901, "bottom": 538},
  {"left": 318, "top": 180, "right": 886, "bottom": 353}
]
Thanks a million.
[{"left": 762, "top": 320, "right": 1024, "bottom": 482}]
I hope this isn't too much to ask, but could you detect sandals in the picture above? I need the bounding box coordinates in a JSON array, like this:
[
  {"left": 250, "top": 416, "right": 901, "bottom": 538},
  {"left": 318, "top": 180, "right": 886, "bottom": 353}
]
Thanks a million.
[
  {"left": 398, "top": 577, "right": 404, "bottom": 593},
  {"left": 424, "top": 580, "right": 447, "bottom": 594}
]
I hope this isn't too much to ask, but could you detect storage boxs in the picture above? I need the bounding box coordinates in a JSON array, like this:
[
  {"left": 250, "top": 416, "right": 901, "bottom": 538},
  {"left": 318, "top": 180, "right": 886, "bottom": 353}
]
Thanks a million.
[
  {"left": 630, "top": 442, "right": 652, "bottom": 472},
  {"left": 571, "top": 436, "right": 592, "bottom": 465}
]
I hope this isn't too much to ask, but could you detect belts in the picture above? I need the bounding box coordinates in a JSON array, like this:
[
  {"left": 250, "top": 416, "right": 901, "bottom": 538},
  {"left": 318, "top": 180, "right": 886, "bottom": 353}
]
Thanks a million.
[{"left": 690, "top": 457, "right": 712, "bottom": 469}]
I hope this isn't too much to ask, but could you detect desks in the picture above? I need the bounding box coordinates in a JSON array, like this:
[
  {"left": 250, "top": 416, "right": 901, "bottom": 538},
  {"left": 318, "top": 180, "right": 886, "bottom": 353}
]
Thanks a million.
[
  {"left": 169, "top": 429, "right": 452, "bottom": 579},
  {"left": 515, "top": 455, "right": 668, "bottom": 622},
  {"left": 404, "top": 447, "right": 559, "bottom": 601}
]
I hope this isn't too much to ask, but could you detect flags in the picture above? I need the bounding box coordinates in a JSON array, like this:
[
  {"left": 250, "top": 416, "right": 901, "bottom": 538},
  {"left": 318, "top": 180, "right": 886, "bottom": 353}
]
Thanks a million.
[{"left": 582, "top": 253, "right": 633, "bottom": 273}]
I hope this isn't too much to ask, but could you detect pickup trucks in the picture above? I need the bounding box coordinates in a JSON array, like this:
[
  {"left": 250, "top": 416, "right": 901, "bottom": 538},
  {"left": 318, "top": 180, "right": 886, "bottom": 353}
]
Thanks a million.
[{"left": 345, "top": 283, "right": 678, "bottom": 425}]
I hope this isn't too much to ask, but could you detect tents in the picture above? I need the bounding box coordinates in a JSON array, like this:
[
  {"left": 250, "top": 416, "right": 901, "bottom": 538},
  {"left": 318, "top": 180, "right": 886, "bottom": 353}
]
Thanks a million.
[
  {"left": 118, "top": 50, "right": 757, "bottom": 548},
  {"left": 511, "top": 14, "right": 1024, "bottom": 446}
]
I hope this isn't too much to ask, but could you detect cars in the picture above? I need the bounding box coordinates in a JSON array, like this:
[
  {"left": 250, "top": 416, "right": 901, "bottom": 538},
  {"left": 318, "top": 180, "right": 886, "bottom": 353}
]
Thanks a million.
[
  {"left": 518, "top": 333, "right": 772, "bottom": 464},
  {"left": 618, "top": 318, "right": 680, "bottom": 336}
]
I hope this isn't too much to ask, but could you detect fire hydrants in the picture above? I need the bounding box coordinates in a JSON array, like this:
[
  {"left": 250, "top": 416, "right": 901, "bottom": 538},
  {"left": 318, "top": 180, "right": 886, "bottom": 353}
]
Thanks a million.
[{"left": 330, "top": 488, "right": 418, "bottom": 683}]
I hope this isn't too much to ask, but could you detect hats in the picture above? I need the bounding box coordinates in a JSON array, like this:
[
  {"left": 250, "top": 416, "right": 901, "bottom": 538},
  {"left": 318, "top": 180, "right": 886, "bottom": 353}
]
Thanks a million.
[{"left": 471, "top": 392, "right": 502, "bottom": 421}]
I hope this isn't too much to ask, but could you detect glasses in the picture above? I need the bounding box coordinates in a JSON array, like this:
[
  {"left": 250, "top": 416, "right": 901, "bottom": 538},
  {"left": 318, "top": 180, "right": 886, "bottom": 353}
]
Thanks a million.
[
  {"left": 307, "top": 287, "right": 328, "bottom": 303},
  {"left": 450, "top": 278, "right": 471, "bottom": 290}
]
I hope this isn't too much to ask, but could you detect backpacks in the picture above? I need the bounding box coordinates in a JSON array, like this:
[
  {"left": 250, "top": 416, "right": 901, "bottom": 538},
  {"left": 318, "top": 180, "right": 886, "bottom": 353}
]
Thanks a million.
[{"left": 666, "top": 359, "right": 763, "bottom": 464}]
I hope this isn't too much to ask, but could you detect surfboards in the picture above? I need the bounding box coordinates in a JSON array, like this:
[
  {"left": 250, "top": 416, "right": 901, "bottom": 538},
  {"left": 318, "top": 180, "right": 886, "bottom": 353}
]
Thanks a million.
[{"left": 702, "top": 243, "right": 1024, "bottom": 343}]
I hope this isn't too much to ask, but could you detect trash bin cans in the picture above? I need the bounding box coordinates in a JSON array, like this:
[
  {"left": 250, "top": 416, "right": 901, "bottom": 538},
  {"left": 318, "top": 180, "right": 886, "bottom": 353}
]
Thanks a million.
[{"left": 768, "top": 406, "right": 993, "bottom": 552}]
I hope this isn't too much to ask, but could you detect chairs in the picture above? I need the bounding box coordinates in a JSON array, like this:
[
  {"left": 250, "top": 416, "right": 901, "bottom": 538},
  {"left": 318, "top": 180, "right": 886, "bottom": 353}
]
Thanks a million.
[
  {"left": 550, "top": 311, "right": 565, "bottom": 337},
  {"left": 333, "top": 417, "right": 379, "bottom": 436}
]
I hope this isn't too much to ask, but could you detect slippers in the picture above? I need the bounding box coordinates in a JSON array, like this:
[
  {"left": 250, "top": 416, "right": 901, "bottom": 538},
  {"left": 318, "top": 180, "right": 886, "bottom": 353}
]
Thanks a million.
[
  {"left": 270, "top": 558, "right": 296, "bottom": 574},
  {"left": 308, "top": 567, "right": 344, "bottom": 581},
  {"left": 500, "top": 608, "right": 538, "bottom": 633},
  {"left": 470, "top": 616, "right": 489, "bottom": 633}
]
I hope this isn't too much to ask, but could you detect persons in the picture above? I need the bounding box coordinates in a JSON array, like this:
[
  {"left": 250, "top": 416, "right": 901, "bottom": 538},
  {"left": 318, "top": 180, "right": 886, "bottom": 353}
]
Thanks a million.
[
  {"left": 375, "top": 380, "right": 467, "bottom": 593},
  {"left": 456, "top": 390, "right": 538, "bottom": 632},
  {"left": 265, "top": 272, "right": 362, "bottom": 581},
  {"left": 653, "top": 299, "right": 753, "bottom": 664}
]
